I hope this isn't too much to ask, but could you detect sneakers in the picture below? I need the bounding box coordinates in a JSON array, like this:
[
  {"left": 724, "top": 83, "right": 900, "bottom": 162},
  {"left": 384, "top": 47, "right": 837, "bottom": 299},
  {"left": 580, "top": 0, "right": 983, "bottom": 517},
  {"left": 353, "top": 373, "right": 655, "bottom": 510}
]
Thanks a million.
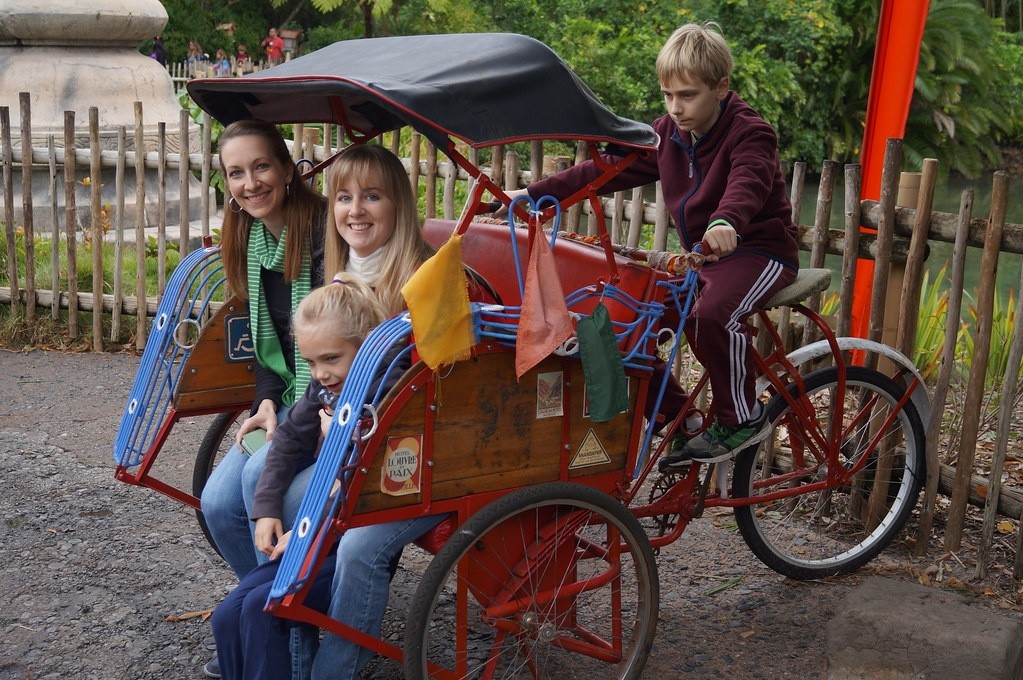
[
  {"left": 658, "top": 427, "right": 693, "bottom": 474},
  {"left": 203, "top": 656, "right": 221, "bottom": 678},
  {"left": 682, "top": 398, "right": 772, "bottom": 463}
]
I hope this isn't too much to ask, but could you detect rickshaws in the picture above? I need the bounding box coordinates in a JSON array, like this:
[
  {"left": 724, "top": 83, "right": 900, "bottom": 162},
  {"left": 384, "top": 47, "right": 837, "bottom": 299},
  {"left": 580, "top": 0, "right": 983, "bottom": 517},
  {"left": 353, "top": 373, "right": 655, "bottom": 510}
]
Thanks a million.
[{"left": 109, "top": 31, "right": 938, "bottom": 677}]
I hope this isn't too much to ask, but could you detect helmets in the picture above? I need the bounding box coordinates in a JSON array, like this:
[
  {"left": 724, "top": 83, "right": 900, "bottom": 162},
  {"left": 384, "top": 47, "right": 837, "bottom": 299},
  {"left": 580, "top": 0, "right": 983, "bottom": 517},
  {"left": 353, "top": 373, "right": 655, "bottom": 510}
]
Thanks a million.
[{"left": 237, "top": 45, "right": 246, "bottom": 50}]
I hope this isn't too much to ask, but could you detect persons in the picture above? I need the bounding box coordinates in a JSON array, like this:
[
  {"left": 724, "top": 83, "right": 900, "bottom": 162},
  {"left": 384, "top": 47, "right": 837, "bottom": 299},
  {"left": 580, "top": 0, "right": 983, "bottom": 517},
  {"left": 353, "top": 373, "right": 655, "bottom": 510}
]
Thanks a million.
[
  {"left": 260, "top": 26, "right": 287, "bottom": 65},
  {"left": 185, "top": 40, "right": 209, "bottom": 66},
  {"left": 199, "top": 117, "right": 359, "bottom": 680},
  {"left": 209, "top": 269, "right": 406, "bottom": 680},
  {"left": 211, "top": 48, "right": 230, "bottom": 75},
  {"left": 255, "top": 139, "right": 479, "bottom": 680},
  {"left": 490, "top": 22, "right": 801, "bottom": 474},
  {"left": 150, "top": 35, "right": 167, "bottom": 70},
  {"left": 235, "top": 43, "right": 253, "bottom": 72}
]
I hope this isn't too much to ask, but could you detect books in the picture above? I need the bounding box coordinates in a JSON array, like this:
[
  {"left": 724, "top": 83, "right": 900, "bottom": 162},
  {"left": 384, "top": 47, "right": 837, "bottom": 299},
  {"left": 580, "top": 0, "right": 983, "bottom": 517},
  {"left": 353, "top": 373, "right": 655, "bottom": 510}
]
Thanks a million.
[{"left": 238, "top": 425, "right": 270, "bottom": 460}]
